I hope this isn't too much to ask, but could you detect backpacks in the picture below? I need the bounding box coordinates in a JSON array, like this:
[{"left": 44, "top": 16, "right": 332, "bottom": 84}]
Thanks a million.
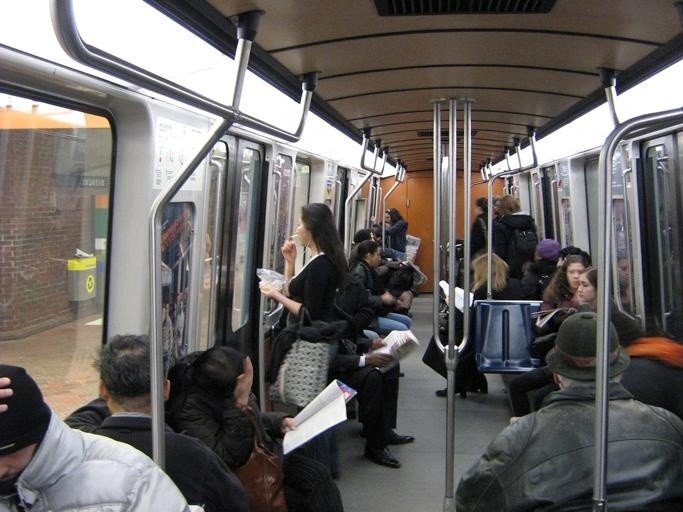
[{"left": 511, "top": 225, "right": 537, "bottom": 255}]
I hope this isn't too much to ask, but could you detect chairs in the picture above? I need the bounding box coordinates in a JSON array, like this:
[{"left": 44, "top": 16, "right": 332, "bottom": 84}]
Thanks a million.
[{"left": 474, "top": 299, "right": 542, "bottom": 374}]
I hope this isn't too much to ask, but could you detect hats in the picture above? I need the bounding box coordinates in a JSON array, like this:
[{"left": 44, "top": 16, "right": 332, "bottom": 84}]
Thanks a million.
[
  {"left": 0, "top": 364, "right": 51, "bottom": 456},
  {"left": 545, "top": 312, "right": 631, "bottom": 381},
  {"left": 536, "top": 238, "right": 561, "bottom": 260}
]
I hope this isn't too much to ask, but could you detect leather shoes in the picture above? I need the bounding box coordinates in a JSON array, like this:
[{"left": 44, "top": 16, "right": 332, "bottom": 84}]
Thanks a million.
[
  {"left": 363, "top": 442, "right": 402, "bottom": 469},
  {"left": 385, "top": 430, "right": 415, "bottom": 445}
]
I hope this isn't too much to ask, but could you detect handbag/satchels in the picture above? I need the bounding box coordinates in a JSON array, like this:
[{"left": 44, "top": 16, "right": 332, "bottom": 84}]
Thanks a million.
[
  {"left": 267, "top": 337, "right": 332, "bottom": 409},
  {"left": 228, "top": 446, "right": 288, "bottom": 511}
]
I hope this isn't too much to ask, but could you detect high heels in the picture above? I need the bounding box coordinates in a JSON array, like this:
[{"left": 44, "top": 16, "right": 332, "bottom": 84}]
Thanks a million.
[
  {"left": 466, "top": 374, "right": 488, "bottom": 394},
  {"left": 435, "top": 383, "right": 468, "bottom": 399}
]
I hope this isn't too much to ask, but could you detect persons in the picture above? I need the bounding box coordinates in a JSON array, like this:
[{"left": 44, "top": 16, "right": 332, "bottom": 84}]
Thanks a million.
[
  {"left": 416, "top": 195, "right": 588, "bottom": 399},
  {"left": 0, "top": 363, "right": 192, "bottom": 512},
  {"left": 457, "top": 310, "right": 683, "bottom": 511},
  {"left": 510, "top": 266, "right": 597, "bottom": 416},
  {"left": 329, "top": 206, "right": 415, "bottom": 469},
  {"left": 173, "top": 344, "right": 345, "bottom": 512},
  {"left": 611, "top": 311, "right": 683, "bottom": 421},
  {"left": 59, "top": 332, "right": 249, "bottom": 512},
  {"left": 259, "top": 202, "right": 350, "bottom": 479}
]
